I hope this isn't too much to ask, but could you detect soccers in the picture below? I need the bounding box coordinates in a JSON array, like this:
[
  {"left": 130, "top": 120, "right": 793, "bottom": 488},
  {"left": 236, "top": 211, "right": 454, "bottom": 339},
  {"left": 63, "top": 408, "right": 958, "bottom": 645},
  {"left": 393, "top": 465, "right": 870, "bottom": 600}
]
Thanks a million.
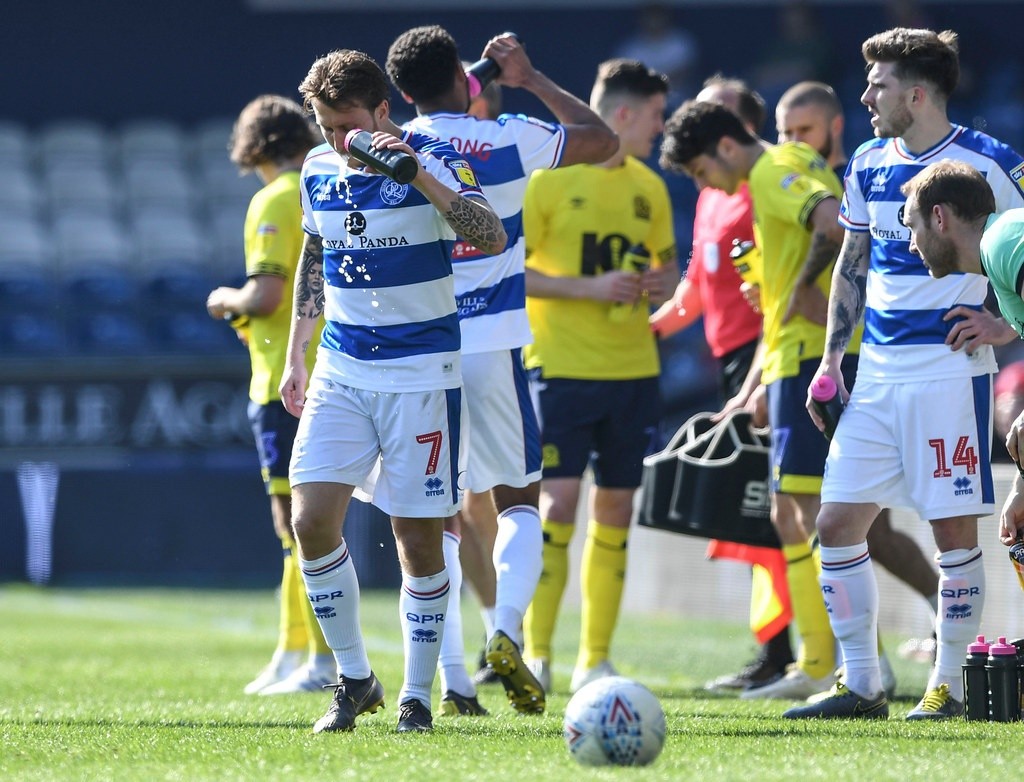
[{"left": 564, "top": 677, "right": 667, "bottom": 768}]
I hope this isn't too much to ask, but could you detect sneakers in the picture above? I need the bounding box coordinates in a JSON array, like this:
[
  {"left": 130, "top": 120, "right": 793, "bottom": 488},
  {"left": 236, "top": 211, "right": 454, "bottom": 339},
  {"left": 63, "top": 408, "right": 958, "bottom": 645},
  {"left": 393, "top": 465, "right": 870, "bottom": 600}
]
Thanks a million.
[
  {"left": 312, "top": 670, "right": 385, "bottom": 734},
  {"left": 394, "top": 698, "right": 433, "bottom": 734},
  {"left": 904, "top": 684, "right": 964, "bottom": 720},
  {"left": 783, "top": 684, "right": 888, "bottom": 720}
]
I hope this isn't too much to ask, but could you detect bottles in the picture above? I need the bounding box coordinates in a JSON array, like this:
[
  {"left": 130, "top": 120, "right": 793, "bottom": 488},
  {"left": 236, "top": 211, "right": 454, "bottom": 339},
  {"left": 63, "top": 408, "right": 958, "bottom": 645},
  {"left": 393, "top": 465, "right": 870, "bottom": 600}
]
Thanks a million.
[
  {"left": 811, "top": 376, "right": 844, "bottom": 439},
  {"left": 729, "top": 238, "right": 761, "bottom": 288},
  {"left": 608, "top": 241, "right": 650, "bottom": 324},
  {"left": 464, "top": 31, "right": 526, "bottom": 103},
  {"left": 1008, "top": 535, "right": 1024, "bottom": 592},
  {"left": 962, "top": 635, "right": 1024, "bottom": 723},
  {"left": 344, "top": 125, "right": 418, "bottom": 184},
  {"left": 224, "top": 310, "right": 251, "bottom": 349}
]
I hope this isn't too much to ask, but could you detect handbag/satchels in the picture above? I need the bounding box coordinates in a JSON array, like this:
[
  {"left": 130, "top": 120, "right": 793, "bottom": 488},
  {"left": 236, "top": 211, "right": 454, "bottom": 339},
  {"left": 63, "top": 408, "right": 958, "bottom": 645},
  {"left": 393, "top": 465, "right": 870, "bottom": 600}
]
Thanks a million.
[{"left": 637, "top": 406, "right": 782, "bottom": 549}]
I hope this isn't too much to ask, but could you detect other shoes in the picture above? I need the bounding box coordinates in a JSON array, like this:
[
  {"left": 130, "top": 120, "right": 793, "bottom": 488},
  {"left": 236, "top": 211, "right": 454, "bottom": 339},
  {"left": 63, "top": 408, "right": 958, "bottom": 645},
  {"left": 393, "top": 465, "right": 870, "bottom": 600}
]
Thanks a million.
[
  {"left": 439, "top": 690, "right": 490, "bottom": 717},
  {"left": 244, "top": 649, "right": 339, "bottom": 697},
  {"left": 568, "top": 661, "right": 619, "bottom": 693},
  {"left": 739, "top": 664, "right": 837, "bottom": 699},
  {"left": 486, "top": 630, "right": 545, "bottom": 714},
  {"left": 703, "top": 651, "right": 787, "bottom": 692},
  {"left": 523, "top": 659, "right": 550, "bottom": 692}
]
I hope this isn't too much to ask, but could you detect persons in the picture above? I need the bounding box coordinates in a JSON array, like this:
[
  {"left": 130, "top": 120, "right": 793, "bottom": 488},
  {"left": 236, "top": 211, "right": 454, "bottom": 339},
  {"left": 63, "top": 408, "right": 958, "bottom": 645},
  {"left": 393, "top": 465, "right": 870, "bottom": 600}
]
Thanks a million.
[
  {"left": 650, "top": 25, "right": 1024, "bottom": 720},
  {"left": 207, "top": 27, "right": 619, "bottom": 734},
  {"left": 519, "top": 57, "right": 680, "bottom": 690}
]
